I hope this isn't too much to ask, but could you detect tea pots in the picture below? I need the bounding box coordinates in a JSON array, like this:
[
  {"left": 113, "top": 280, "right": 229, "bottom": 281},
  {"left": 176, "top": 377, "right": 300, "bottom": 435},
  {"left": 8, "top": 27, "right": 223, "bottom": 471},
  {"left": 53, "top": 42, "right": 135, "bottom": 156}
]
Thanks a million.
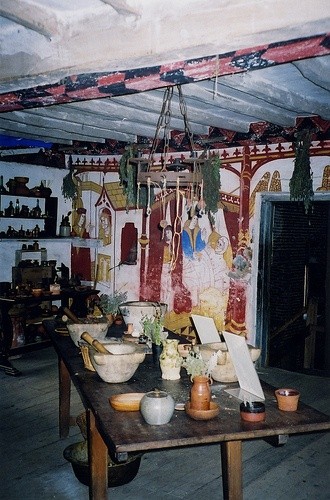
[{"left": 159, "top": 338, "right": 183, "bottom": 380}]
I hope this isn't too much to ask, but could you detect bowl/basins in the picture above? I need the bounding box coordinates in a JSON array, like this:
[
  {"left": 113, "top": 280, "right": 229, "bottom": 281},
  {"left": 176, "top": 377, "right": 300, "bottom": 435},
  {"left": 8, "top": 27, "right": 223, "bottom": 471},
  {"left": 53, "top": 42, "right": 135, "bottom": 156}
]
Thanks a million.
[
  {"left": 178, "top": 343, "right": 191, "bottom": 357},
  {"left": 185, "top": 401, "right": 220, "bottom": 421},
  {"left": 111, "top": 393, "right": 145, "bottom": 411}
]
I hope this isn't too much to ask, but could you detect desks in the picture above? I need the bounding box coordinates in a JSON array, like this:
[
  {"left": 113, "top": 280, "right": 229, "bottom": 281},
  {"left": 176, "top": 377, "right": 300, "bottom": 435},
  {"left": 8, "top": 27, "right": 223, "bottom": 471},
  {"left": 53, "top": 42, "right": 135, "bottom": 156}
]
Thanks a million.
[
  {"left": 41, "top": 316, "right": 313, "bottom": 500},
  {"left": 0, "top": 284, "right": 101, "bottom": 377}
]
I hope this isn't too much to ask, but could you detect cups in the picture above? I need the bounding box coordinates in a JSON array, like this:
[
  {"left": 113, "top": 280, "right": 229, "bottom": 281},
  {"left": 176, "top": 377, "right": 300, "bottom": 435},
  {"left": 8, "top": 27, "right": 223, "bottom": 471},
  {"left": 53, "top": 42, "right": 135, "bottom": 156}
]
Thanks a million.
[
  {"left": 41, "top": 261, "right": 48, "bottom": 267},
  {"left": 275, "top": 389, "right": 300, "bottom": 411},
  {"left": 240, "top": 400, "right": 266, "bottom": 421}
]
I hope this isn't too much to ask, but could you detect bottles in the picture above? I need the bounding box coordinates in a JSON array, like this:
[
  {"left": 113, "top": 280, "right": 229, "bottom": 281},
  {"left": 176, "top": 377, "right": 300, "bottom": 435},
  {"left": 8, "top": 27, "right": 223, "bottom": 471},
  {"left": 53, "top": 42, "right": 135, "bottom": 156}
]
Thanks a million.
[
  {"left": 6, "top": 224, "right": 40, "bottom": 238},
  {"left": 191, "top": 376, "right": 211, "bottom": 410},
  {"left": 140, "top": 391, "right": 174, "bottom": 425},
  {"left": 22, "top": 240, "right": 38, "bottom": 251},
  {"left": 33, "top": 199, "right": 42, "bottom": 217},
  {"left": 8, "top": 201, "right": 14, "bottom": 217},
  {"left": 26, "top": 260, "right": 39, "bottom": 267},
  {"left": 15, "top": 199, "right": 19, "bottom": 217}
]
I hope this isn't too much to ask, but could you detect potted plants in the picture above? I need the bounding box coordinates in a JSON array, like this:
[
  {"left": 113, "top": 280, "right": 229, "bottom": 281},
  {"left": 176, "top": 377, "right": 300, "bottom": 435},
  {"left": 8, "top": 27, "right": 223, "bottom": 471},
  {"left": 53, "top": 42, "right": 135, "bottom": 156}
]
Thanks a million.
[
  {"left": 142, "top": 302, "right": 169, "bottom": 364},
  {"left": 96, "top": 290, "right": 128, "bottom": 338}
]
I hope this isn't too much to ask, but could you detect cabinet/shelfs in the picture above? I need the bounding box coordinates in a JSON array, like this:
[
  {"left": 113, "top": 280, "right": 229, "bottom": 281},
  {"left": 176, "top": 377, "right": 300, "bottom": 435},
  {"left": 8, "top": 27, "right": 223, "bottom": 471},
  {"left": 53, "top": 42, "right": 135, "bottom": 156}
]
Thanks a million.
[{"left": 0, "top": 194, "right": 58, "bottom": 238}]
